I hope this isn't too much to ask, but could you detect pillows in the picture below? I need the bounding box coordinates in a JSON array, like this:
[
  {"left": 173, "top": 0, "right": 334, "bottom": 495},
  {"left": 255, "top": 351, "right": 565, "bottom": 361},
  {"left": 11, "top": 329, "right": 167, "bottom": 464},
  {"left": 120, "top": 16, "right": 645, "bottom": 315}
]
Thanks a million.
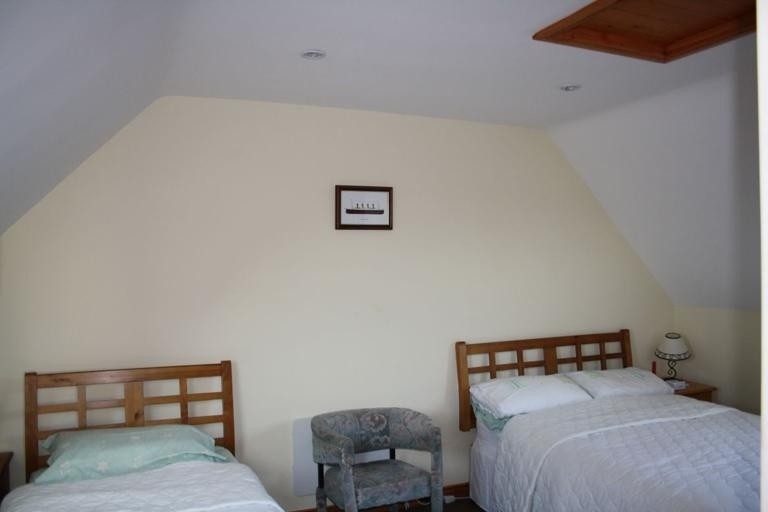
[
  {"left": 468, "top": 365, "right": 676, "bottom": 418},
  {"left": 29, "top": 423, "right": 232, "bottom": 484}
]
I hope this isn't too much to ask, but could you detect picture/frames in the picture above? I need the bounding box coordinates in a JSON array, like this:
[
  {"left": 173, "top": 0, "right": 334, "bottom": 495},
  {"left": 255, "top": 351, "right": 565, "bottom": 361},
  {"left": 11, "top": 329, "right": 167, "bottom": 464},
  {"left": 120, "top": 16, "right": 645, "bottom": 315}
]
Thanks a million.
[{"left": 336, "top": 186, "right": 393, "bottom": 230}]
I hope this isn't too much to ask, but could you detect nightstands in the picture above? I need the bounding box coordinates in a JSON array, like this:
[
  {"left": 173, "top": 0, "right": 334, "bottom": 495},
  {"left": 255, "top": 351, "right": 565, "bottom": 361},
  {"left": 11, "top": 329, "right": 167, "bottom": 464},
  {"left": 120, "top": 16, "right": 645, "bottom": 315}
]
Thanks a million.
[{"left": 672, "top": 379, "right": 716, "bottom": 402}]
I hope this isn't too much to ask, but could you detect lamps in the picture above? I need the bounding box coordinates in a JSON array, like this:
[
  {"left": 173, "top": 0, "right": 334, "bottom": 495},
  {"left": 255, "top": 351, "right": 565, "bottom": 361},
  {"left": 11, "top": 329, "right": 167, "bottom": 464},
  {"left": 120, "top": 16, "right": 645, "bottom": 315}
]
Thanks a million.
[{"left": 655, "top": 330, "right": 690, "bottom": 381}]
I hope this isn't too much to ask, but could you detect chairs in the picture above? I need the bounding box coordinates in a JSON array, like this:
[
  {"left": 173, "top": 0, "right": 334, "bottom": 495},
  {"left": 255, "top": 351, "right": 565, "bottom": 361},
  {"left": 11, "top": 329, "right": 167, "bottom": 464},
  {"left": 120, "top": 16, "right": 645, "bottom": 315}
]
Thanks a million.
[{"left": 311, "top": 408, "right": 444, "bottom": 512}]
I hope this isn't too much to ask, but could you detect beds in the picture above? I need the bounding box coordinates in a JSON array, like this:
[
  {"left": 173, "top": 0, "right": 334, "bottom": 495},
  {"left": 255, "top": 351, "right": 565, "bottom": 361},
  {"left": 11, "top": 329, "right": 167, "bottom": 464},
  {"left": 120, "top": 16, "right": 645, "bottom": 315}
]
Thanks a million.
[
  {"left": 454, "top": 329, "right": 761, "bottom": 512},
  {"left": 0, "top": 358, "right": 282, "bottom": 512}
]
